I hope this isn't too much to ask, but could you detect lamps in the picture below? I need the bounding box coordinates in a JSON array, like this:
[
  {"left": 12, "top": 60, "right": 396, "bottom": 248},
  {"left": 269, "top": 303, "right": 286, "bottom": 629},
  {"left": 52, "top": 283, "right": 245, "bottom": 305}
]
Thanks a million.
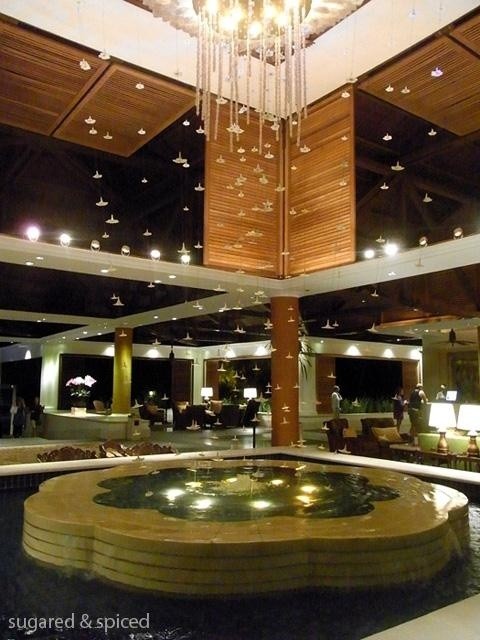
[
  {"left": 201, "top": 387, "right": 213, "bottom": 410},
  {"left": 125, "top": 0, "right": 373, "bottom": 155},
  {"left": 364, "top": 228, "right": 465, "bottom": 259},
  {"left": 428, "top": 403, "right": 457, "bottom": 453},
  {"left": 457, "top": 404, "right": 480, "bottom": 455},
  {"left": 244, "top": 388, "right": 257, "bottom": 401},
  {"left": 16, "top": 226, "right": 191, "bottom": 265}
]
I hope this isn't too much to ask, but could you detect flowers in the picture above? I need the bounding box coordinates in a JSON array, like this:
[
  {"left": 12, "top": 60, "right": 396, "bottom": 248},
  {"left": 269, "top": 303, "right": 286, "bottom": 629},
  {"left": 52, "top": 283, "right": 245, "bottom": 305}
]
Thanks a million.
[{"left": 66, "top": 375, "right": 97, "bottom": 396}]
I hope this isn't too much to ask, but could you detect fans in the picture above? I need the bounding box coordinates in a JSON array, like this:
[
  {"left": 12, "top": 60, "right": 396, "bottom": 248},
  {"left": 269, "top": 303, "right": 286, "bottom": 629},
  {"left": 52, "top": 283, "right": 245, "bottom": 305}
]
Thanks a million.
[{"left": 430, "top": 328, "right": 476, "bottom": 347}]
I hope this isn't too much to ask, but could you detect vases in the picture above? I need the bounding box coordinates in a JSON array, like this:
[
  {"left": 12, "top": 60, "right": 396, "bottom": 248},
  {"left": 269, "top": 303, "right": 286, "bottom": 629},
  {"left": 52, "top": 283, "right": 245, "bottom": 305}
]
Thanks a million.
[{"left": 72, "top": 397, "right": 88, "bottom": 407}]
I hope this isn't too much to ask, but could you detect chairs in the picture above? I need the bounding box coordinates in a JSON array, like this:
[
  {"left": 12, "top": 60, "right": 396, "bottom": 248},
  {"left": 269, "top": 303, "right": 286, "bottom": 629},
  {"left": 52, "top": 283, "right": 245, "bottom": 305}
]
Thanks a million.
[
  {"left": 323, "top": 418, "right": 381, "bottom": 459},
  {"left": 139, "top": 400, "right": 260, "bottom": 430},
  {"left": 361, "top": 418, "right": 414, "bottom": 455}
]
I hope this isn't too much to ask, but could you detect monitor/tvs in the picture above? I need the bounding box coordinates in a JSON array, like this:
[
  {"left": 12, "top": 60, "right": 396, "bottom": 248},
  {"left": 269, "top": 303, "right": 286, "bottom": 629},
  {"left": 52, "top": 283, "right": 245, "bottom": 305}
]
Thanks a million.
[{"left": 446, "top": 389, "right": 461, "bottom": 402}]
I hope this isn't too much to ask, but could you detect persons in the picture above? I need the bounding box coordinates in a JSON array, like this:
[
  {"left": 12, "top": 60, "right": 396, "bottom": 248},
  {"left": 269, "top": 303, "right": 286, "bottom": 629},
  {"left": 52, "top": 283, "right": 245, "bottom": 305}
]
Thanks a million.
[
  {"left": 29, "top": 398, "right": 42, "bottom": 436},
  {"left": 408, "top": 383, "right": 428, "bottom": 437},
  {"left": 436, "top": 385, "right": 449, "bottom": 402},
  {"left": 392, "top": 387, "right": 408, "bottom": 432},
  {"left": 12, "top": 399, "right": 27, "bottom": 438},
  {"left": 332, "top": 385, "right": 342, "bottom": 418},
  {"left": 147, "top": 399, "right": 171, "bottom": 425}
]
